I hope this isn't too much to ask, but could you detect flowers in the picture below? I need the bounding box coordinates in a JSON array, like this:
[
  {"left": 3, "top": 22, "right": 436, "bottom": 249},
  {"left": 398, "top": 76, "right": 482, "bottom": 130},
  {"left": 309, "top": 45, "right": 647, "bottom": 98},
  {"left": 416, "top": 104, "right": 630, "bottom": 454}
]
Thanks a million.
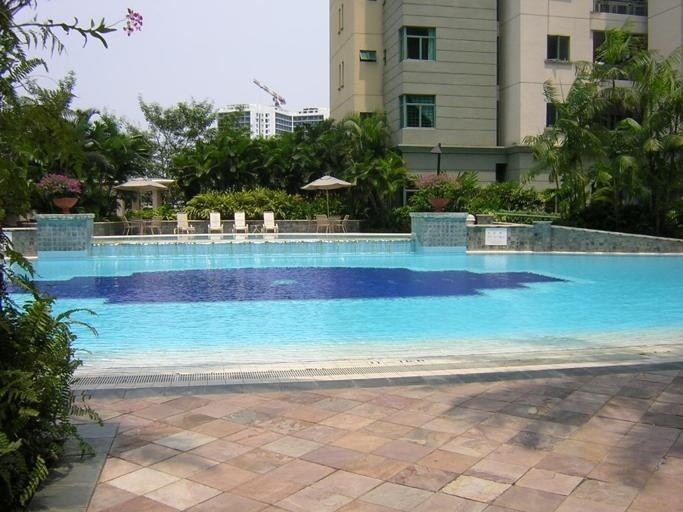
[
  {"left": 417, "top": 174, "right": 461, "bottom": 199},
  {"left": 39, "top": 174, "right": 81, "bottom": 200}
]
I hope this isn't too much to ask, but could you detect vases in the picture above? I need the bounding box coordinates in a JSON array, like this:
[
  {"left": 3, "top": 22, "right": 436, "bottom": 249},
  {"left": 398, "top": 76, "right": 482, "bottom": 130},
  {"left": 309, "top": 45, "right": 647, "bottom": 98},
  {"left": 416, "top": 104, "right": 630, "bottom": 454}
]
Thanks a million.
[
  {"left": 428, "top": 199, "right": 449, "bottom": 209},
  {"left": 53, "top": 198, "right": 78, "bottom": 215}
]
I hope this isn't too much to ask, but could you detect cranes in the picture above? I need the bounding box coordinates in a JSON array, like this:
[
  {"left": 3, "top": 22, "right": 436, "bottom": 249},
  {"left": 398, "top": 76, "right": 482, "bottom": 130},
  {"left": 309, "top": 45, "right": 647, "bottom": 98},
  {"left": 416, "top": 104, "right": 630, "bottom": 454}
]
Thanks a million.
[{"left": 251, "top": 77, "right": 288, "bottom": 108}]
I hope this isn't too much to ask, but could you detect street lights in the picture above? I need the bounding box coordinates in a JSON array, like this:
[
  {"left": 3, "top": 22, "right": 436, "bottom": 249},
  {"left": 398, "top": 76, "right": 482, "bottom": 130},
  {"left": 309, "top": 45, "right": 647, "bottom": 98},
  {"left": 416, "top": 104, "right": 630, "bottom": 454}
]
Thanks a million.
[{"left": 430, "top": 141, "right": 443, "bottom": 179}]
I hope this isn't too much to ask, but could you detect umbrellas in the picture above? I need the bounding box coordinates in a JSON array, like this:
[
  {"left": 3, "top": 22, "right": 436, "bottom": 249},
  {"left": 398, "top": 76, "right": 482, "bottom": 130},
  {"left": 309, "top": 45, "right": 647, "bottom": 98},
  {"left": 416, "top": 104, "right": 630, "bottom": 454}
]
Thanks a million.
[
  {"left": 112, "top": 178, "right": 167, "bottom": 234},
  {"left": 298, "top": 174, "right": 352, "bottom": 219}
]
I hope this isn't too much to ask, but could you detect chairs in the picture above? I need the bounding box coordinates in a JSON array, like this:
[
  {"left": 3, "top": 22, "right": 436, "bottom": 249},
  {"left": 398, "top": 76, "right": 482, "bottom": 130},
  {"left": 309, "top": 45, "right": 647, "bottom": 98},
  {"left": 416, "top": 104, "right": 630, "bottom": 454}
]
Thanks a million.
[{"left": 117, "top": 203, "right": 350, "bottom": 235}]
